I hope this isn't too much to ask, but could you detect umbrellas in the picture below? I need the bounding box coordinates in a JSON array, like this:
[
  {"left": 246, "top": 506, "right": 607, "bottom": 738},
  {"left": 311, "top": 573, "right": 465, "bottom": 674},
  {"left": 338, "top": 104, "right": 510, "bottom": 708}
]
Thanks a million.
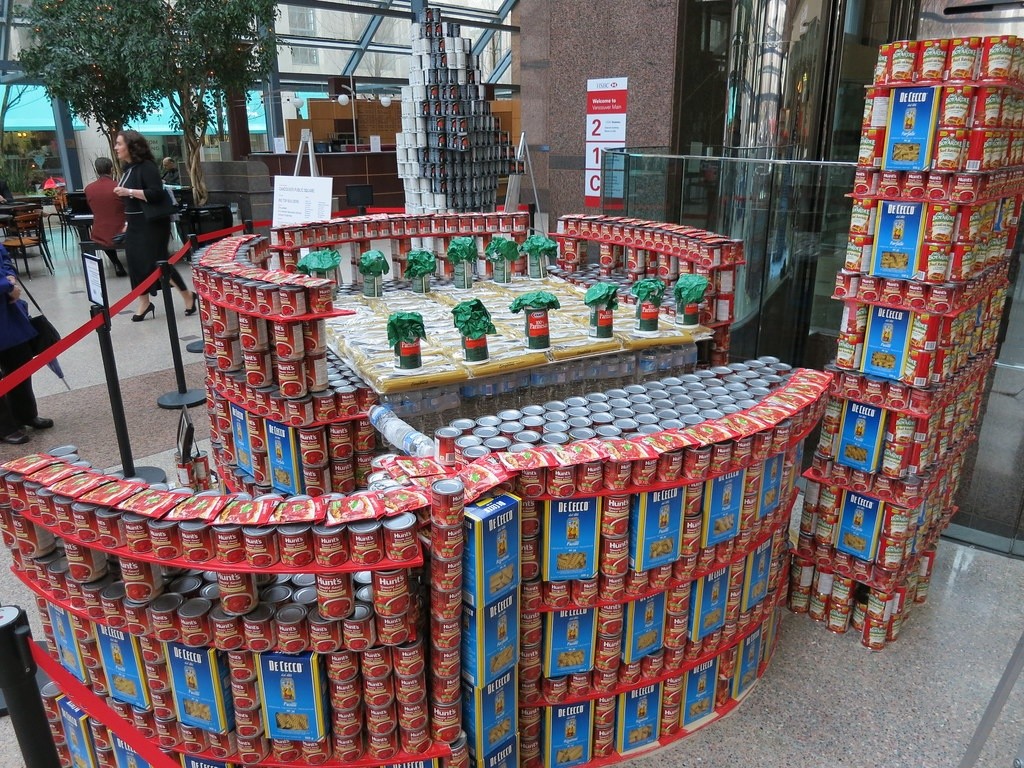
[
  {"left": 38, "top": 176, "right": 66, "bottom": 190},
  {"left": 27, "top": 315, "right": 73, "bottom": 391}
]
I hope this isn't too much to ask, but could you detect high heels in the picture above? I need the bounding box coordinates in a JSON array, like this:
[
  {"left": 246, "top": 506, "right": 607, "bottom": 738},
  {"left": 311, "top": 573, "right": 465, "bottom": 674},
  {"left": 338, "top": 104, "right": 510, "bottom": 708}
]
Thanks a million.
[
  {"left": 184, "top": 291, "right": 198, "bottom": 316},
  {"left": 130, "top": 301, "right": 155, "bottom": 322}
]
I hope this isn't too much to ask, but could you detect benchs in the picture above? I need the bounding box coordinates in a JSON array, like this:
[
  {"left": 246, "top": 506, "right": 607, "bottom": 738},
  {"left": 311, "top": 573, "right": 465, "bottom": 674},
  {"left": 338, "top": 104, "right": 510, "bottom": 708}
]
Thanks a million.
[{"left": 78, "top": 240, "right": 126, "bottom": 258}]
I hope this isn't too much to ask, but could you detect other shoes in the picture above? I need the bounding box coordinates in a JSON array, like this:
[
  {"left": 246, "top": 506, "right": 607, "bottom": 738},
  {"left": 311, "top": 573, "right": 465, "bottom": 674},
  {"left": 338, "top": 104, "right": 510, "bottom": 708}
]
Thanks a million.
[{"left": 113, "top": 262, "right": 127, "bottom": 277}]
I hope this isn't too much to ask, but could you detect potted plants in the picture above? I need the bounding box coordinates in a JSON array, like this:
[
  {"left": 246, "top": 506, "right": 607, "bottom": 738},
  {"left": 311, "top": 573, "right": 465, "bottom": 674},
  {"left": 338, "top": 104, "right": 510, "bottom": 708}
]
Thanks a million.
[{"left": 0, "top": 0, "right": 296, "bottom": 255}]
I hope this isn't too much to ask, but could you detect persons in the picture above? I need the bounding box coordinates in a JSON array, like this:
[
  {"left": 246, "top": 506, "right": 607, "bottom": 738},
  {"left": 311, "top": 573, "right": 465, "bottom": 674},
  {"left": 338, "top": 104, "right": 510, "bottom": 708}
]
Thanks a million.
[
  {"left": 0, "top": 178, "right": 18, "bottom": 237},
  {"left": 0, "top": 243, "right": 53, "bottom": 444},
  {"left": 161, "top": 157, "right": 180, "bottom": 186},
  {"left": 113, "top": 130, "right": 198, "bottom": 322},
  {"left": 85, "top": 157, "right": 128, "bottom": 277},
  {"left": 56, "top": 186, "right": 69, "bottom": 224}
]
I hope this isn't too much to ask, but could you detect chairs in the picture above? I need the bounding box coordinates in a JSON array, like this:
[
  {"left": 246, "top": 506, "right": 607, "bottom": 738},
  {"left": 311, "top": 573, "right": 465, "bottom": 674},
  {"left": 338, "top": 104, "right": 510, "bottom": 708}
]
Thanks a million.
[{"left": 0, "top": 194, "right": 79, "bottom": 279}]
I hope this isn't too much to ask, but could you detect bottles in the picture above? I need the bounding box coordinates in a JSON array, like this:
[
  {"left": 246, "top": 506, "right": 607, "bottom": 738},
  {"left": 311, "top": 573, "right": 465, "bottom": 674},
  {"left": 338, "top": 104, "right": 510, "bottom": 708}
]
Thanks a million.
[{"left": 369, "top": 342, "right": 697, "bottom": 457}]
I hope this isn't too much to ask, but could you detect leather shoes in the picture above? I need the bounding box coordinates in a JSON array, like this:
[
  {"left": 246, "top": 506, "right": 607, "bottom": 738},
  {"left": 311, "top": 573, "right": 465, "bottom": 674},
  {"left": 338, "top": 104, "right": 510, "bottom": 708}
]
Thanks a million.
[
  {"left": 0, "top": 430, "right": 29, "bottom": 443},
  {"left": 25, "top": 415, "right": 53, "bottom": 429}
]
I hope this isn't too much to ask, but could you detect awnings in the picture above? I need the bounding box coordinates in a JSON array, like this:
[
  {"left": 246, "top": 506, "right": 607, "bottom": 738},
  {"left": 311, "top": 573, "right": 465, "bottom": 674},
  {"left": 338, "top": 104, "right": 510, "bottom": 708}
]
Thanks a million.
[
  {"left": 123, "top": 89, "right": 329, "bottom": 136},
  {"left": 0, "top": 85, "right": 87, "bottom": 132}
]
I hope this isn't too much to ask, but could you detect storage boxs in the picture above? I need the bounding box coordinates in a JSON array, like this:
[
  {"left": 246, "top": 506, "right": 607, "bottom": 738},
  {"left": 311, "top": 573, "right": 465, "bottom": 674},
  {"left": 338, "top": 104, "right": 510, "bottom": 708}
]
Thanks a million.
[
  {"left": 94, "top": 624, "right": 154, "bottom": 709},
  {"left": 881, "top": 86, "right": 942, "bottom": 172},
  {"left": 869, "top": 201, "right": 928, "bottom": 278},
  {"left": 178, "top": 752, "right": 242, "bottom": 768},
  {"left": 381, "top": 757, "right": 440, "bottom": 768},
  {"left": 832, "top": 489, "right": 886, "bottom": 564},
  {"left": 54, "top": 693, "right": 100, "bottom": 768},
  {"left": 860, "top": 303, "right": 915, "bottom": 381},
  {"left": 834, "top": 400, "right": 891, "bottom": 476},
  {"left": 226, "top": 401, "right": 256, "bottom": 476},
  {"left": 106, "top": 729, "right": 149, "bottom": 768},
  {"left": 459, "top": 451, "right": 787, "bottom": 768},
  {"left": 43, "top": 598, "right": 92, "bottom": 687},
  {"left": 165, "top": 641, "right": 236, "bottom": 736},
  {"left": 262, "top": 420, "right": 307, "bottom": 496},
  {"left": 254, "top": 653, "right": 331, "bottom": 742}
]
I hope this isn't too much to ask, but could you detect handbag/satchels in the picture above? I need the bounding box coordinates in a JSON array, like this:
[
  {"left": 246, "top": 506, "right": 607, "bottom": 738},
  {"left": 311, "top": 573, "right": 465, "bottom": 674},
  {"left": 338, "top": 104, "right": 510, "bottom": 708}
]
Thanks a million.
[
  {"left": 4, "top": 262, "right": 61, "bottom": 356},
  {"left": 137, "top": 165, "right": 183, "bottom": 224}
]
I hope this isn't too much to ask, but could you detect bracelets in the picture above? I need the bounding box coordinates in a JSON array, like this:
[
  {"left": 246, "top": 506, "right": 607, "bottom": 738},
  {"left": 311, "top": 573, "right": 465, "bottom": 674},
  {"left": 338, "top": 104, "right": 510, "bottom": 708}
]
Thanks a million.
[{"left": 129, "top": 189, "right": 133, "bottom": 199}]
